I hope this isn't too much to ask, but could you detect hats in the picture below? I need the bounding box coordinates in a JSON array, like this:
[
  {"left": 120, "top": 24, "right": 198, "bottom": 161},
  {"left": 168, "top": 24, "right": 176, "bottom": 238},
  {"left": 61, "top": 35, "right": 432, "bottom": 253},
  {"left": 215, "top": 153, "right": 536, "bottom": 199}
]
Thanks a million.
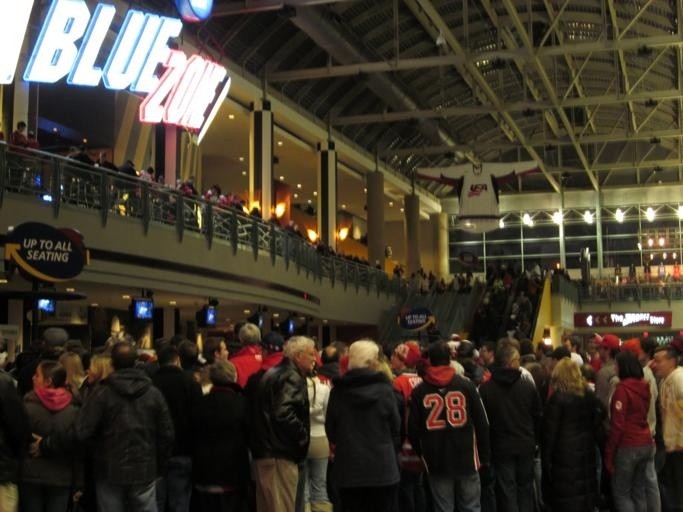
[
  {"left": 258, "top": 332, "right": 284, "bottom": 352},
  {"left": 394, "top": 343, "right": 421, "bottom": 369},
  {"left": 620, "top": 338, "right": 641, "bottom": 357},
  {"left": 593, "top": 334, "right": 620, "bottom": 348},
  {"left": 43, "top": 328, "right": 69, "bottom": 346},
  {"left": 68, "top": 340, "right": 88, "bottom": 355},
  {"left": 546, "top": 346, "right": 571, "bottom": 360}
]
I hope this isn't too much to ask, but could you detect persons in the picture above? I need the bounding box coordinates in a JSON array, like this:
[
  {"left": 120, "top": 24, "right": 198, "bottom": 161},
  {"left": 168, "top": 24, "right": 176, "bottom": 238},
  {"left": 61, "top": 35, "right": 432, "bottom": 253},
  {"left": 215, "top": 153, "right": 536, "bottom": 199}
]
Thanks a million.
[{"left": 1, "top": 120, "right": 683, "bottom": 512}]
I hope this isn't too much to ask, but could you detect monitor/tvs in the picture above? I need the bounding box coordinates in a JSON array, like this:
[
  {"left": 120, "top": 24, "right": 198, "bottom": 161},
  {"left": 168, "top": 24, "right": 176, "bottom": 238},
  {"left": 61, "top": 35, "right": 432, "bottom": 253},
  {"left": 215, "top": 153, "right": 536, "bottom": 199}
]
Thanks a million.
[
  {"left": 38, "top": 295, "right": 57, "bottom": 315},
  {"left": 132, "top": 298, "right": 153, "bottom": 322},
  {"left": 195, "top": 306, "right": 217, "bottom": 327},
  {"left": 249, "top": 312, "right": 265, "bottom": 330},
  {"left": 281, "top": 317, "right": 295, "bottom": 337}
]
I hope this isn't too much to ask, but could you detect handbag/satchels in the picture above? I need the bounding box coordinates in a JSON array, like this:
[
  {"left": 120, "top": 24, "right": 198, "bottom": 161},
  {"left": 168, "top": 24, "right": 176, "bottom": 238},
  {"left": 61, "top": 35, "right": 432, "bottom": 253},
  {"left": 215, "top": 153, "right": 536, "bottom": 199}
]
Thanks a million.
[{"left": 591, "top": 405, "right": 610, "bottom": 439}]
